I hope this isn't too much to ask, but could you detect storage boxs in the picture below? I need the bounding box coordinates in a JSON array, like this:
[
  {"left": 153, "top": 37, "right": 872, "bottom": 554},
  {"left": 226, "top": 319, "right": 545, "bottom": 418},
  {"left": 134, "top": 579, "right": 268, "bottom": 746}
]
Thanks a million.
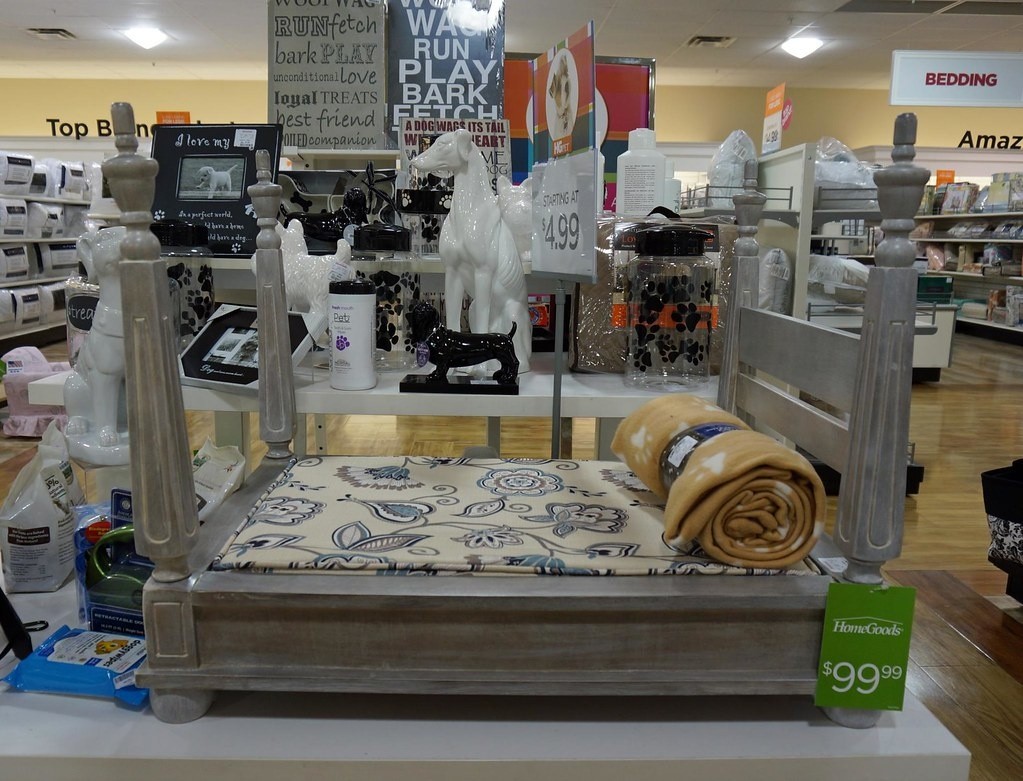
[
  {"left": 918, "top": 275, "right": 954, "bottom": 304},
  {"left": 940, "top": 182, "right": 978, "bottom": 213},
  {"left": 1013, "top": 294, "right": 1023, "bottom": 327},
  {"left": 914, "top": 261, "right": 928, "bottom": 274},
  {"left": 1006, "top": 286, "right": 1023, "bottom": 326}
]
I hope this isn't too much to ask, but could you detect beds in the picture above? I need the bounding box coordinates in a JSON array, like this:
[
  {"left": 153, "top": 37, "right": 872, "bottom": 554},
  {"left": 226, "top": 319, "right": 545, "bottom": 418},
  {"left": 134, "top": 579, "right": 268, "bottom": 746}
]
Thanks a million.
[{"left": 134, "top": 394, "right": 891, "bottom": 726}]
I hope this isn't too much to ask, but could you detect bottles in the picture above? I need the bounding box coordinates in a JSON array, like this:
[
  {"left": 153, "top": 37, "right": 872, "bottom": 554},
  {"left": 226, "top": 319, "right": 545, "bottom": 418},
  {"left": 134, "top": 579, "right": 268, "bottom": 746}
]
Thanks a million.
[
  {"left": 615, "top": 127, "right": 664, "bottom": 217},
  {"left": 664, "top": 160, "right": 682, "bottom": 214}
]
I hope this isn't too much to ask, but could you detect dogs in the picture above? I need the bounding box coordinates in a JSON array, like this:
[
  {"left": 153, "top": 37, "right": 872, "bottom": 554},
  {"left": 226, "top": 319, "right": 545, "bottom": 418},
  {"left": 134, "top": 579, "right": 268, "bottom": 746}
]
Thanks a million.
[
  {"left": 548, "top": 54, "right": 573, "bottom": 136},
  {"left": 251, "top": 218, "right": 355, "bottom": 349},
  {"left": 64, "top": 226, "right": 128, "bottom": 446},
  {"left": 408, "top": 128, "right": 533, "bottom": 378},
  {"left": 496, "top": 176, "right": 533, "bottom": 261},
  {"left": 195, "top": 164, "right": 239, "bottom": 199}
]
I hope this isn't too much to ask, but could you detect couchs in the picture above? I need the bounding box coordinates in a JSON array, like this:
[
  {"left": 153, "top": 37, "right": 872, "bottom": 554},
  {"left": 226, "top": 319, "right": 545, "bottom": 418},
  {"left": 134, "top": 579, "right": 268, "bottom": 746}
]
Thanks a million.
[{"left": 0, "top": 346, "right": 71, "bottom": 437}]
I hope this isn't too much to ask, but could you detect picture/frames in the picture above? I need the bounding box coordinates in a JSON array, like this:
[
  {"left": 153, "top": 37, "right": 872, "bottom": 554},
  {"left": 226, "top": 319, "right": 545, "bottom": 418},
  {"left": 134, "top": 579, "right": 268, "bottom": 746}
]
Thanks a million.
[
  {"left": 148, "top": 124, "right": 283, "bottom": 259},
  {"left": 177, "top": 303, "right": 329, "bottom": 394}
]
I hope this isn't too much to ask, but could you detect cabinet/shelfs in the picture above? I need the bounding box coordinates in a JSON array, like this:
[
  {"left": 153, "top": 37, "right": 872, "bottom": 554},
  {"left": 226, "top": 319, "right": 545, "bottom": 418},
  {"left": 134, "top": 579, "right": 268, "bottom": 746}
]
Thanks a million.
[{"left": 0, "top": 142, "right": 1023, "bottom": 779}]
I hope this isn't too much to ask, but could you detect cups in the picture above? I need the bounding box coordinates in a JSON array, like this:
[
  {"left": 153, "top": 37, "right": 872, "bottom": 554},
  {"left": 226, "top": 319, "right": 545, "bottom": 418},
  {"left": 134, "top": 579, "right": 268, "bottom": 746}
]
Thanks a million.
[{"left": 327, "top": 280, "right": 378, "bottom": 391}]
